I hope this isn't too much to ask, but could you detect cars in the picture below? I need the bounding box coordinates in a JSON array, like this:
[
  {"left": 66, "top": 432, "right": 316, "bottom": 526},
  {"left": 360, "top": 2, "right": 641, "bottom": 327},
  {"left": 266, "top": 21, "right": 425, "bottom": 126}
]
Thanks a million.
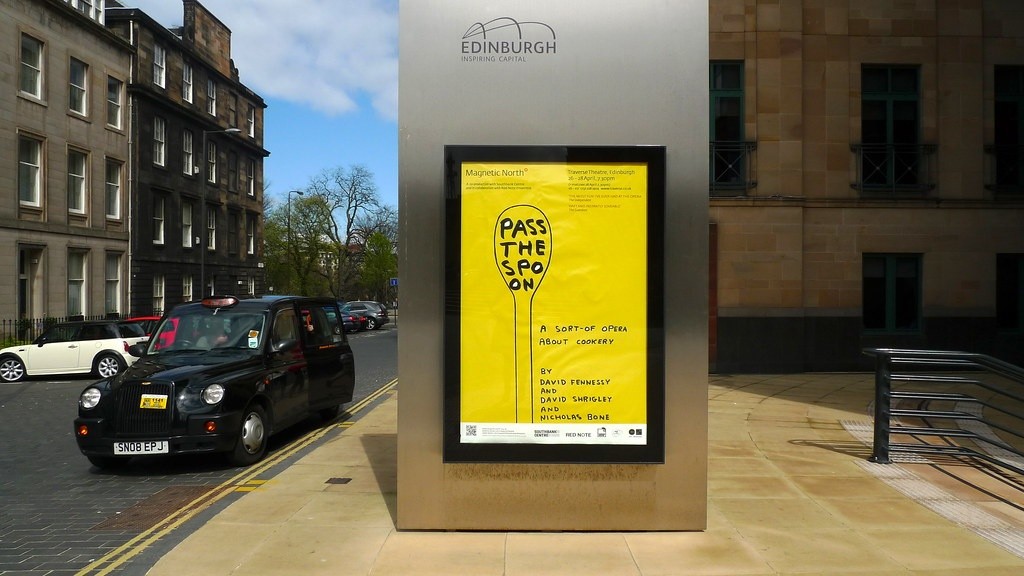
[
  {"left": 300, "top": 299, "right": 390, "bottom": 336},
  {"left": 128, "top": 316, "right": 200, "bottom": 351}
]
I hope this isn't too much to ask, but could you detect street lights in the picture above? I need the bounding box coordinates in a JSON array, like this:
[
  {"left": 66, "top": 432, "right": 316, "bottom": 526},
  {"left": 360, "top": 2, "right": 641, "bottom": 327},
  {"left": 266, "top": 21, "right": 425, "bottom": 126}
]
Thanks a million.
[{"left": 286, "top": 190, "right": 304, "bottom": 295}]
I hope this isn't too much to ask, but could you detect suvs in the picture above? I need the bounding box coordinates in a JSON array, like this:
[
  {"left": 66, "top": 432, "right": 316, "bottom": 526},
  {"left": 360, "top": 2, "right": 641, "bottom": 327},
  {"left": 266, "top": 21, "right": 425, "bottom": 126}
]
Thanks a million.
[
  {"left": 0, "top": 320, "right": 153, "bottom": 383},
  {"left": 72, "top": 294, "right": 356, "bottom": 475}
]
